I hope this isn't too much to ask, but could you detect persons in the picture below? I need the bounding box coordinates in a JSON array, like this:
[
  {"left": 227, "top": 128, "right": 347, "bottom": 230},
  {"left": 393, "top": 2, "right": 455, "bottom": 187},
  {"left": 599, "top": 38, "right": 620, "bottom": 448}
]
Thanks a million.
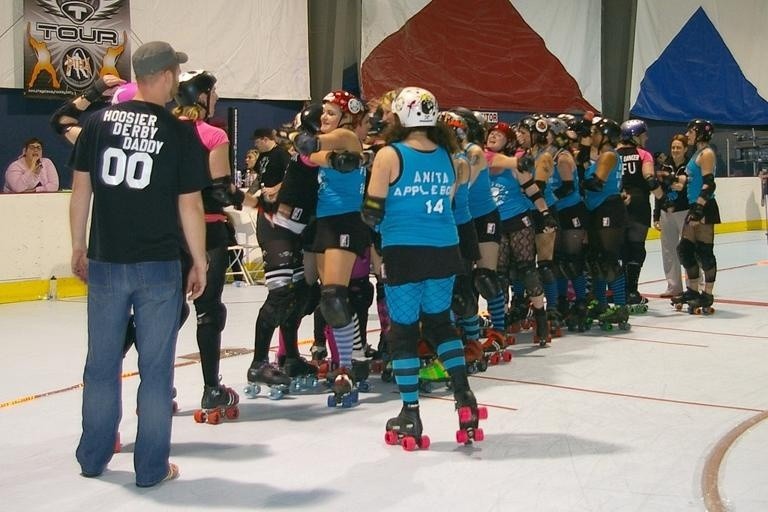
[
  {"left": 240, "top": 86, "right": 631, "bottom": 408},
  {"left": 654, "top": 133, "right": 694, "bottom": 298},
  {"left": 51, "top": 75, "right": 138, "bottom": 454},
  {"left": 366, "top": 87, "right": 487, "bottom": 450},
  {"left": 69, "top": 41, "right": 208, "bottom": 485},
  {"left": 622, "top": 115, "right": 679, "bottom": 312},
  {"left": 138, "top": 67, "right": 240, "bottom": 424},
  {"left": 4, "top": 138, "right": 59, "bottom": 192},
  {"left": 669, "top": 117, "right": 721, "bottom": 314}
]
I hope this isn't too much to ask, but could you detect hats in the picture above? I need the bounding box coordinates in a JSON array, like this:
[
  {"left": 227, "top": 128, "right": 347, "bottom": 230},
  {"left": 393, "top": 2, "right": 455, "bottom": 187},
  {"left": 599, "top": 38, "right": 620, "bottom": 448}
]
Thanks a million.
[
  {"left": 133, "top": 42, "right": 188, "bottom": 75},
  {"left": 247, "top": 149, "right": 260, "bottom": 160}
]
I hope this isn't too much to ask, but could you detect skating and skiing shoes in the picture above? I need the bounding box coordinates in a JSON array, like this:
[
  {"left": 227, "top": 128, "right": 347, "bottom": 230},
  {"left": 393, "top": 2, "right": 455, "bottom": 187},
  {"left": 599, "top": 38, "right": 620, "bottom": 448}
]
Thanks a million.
[
  {"left": 312, "top": 333, "right": 394, "bottom": 407},
  {"left": 464, "top": 329, "right": 515, "bottom": 374},
  {"left": 419, "top": 339, "right": 453, "bottom": 393},
  {"left": 627, "top": 291, "right": 649, "bottom": 315},
  {"left": 244, "top": 360, "right": 290, "bottom": 399},
  {"left": 452, "top": 377, "right": 488, "bottom": 444},
  {"left": 136, "top": 387, "right": 178, "bottom": 416},
  {"left": 506, "top": 295, "right": 630, "bottom": 347},
  {"left": 194, "top": 384, "right": 239, "bottom": 424},
  {"left": 269, "top": 352, "right": 319, "bottom": 393},
  {"left": 385, "top": 401, "right": 430, "bottom": 451},
  {"left": 686, "top": 290, "right": 714, "bottom": 315},
  {"left": 670, "top": 287, "right": 701, "bottom": 310}
]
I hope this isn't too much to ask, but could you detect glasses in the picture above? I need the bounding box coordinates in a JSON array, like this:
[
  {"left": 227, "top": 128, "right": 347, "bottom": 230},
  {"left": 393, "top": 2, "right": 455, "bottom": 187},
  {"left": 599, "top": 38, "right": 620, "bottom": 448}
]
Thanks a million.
[{"left": 30, "top": 146, "right": 42, "bottom": 149}]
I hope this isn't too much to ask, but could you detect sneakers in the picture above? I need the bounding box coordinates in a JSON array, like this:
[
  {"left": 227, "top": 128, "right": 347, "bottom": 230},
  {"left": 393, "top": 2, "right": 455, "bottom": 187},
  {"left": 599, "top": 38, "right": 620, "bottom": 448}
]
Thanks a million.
[
  {"left": 660, "top": 291, "right": 684, "bottom": 297},
  {"left": 161, "top": 463, "right": 180, "bottom": 482}
]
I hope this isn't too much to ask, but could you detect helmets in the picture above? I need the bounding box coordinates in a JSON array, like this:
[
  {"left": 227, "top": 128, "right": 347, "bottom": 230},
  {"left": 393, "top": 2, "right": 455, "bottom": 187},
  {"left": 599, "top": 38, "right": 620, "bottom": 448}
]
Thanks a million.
[
  {"left": 437, "top": 108, "right": 488, "bottom": 145},
  {"left": 301, "top": 90, "right": 364, "bottom": 134},
  {"left": 112, "top": 83, "right": 138, "bottom": 104},
  {"left": 249, "top": 127, "right": 275, "bottom": 140},
  {"left": 622, "top": 120, "right": 647, "bottom": 141},
  {"left": 518, "top": 113, "right": 621, "bottom": 149},
  {"left": 687, "top": 118, "right": 714, "bottom": 141},
  {"left": 378, "top": 86, "right": 438, "bottom": 128},
  {"left": 173, "top": 69, "right": 217, "bottom": 106},
  {"left": 488, "top": 122, "right": 518, "bottom": 156}
]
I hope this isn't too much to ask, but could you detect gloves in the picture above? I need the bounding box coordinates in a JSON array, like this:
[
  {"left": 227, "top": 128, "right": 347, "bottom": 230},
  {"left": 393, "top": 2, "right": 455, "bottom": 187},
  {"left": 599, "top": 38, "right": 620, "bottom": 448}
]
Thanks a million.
[
  {"left": 685, "top": 202, "right": 705, "bottom": 224},
  {"left": 542, "top": 215, "right": 559, "bottom": 233}
]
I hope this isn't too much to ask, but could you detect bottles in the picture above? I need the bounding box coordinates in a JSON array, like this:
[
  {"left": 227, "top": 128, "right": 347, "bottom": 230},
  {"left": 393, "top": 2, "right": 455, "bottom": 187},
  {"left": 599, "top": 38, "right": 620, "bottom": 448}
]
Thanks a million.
[{"left": 245, "top": 170, "right": 251, "bottom": 189}]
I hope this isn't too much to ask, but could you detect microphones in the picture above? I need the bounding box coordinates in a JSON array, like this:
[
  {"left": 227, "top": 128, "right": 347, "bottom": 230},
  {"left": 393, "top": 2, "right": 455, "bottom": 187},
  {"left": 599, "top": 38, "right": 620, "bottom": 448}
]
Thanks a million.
[{"left": 34, "top": 155, "right": 42, "bottom": 167}]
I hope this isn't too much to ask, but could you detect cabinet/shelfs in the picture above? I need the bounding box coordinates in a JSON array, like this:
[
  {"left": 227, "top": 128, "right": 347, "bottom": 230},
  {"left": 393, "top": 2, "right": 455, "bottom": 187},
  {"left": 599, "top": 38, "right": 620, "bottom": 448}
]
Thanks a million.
[
  {"left": 0, "top": 187, "right": 264, "bottom": 304},
  {"left": 645, "top": 177, "right": 768, "bottom": 239},
  {"left": 724, "top": 128, "right": 768, "bottom": 180}
]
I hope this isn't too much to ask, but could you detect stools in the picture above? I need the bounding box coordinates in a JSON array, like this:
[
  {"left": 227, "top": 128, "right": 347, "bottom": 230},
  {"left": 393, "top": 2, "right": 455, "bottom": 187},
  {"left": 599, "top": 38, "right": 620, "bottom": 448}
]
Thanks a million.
[{"left": 222, "top": 244, "right": 261, "bottom": 287}]
